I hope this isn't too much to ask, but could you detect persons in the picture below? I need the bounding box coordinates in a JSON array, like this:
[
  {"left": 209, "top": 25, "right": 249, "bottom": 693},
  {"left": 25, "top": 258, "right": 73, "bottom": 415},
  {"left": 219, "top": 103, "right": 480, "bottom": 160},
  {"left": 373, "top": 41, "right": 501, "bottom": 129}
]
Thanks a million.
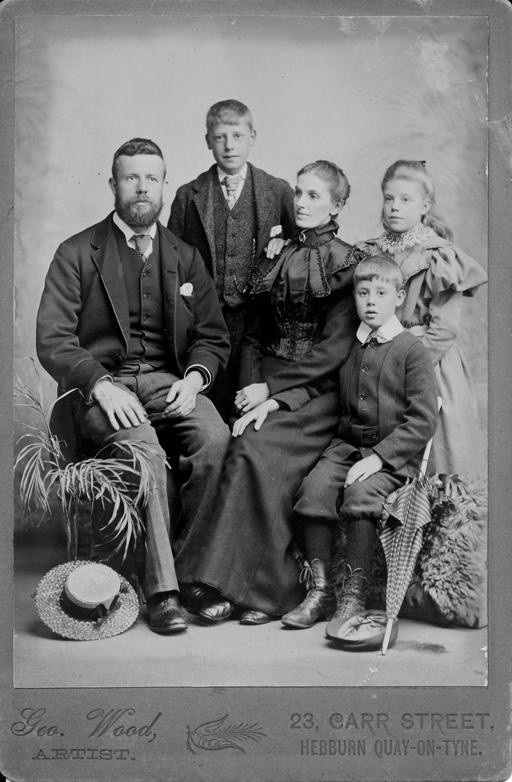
[
  {"left": 231, "top": 253, "right": 439, "bottom": 639},
  {"left": 173, "top": 160, "right": 370, "bottom": 624},
  {"left": 351, "top": 159, "right": 483, "bottom": 484},
  {"left": 35, "top": 136, "right": 230, "bottom": 634},
  {"left": 168, "top": 99, "right": 302, "bottom": 424}
]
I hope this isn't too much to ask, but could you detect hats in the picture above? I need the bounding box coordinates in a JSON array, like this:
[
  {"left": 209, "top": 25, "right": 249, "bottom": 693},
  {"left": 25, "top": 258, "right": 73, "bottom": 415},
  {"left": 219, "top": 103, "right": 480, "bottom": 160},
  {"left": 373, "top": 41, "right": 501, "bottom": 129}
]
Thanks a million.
[
  {"left": 327, "top": 609, "right": 398, "bottom": 652},
  {"left": 38, "top": 561, "right": 140, "bottom": 641}
]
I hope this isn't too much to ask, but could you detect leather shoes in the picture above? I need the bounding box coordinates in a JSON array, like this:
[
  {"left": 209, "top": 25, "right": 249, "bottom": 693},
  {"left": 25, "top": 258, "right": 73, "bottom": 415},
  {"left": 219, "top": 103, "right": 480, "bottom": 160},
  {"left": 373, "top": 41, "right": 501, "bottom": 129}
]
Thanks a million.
[{"left": 147, "top": 581, "right": 271, "bottom": 635}]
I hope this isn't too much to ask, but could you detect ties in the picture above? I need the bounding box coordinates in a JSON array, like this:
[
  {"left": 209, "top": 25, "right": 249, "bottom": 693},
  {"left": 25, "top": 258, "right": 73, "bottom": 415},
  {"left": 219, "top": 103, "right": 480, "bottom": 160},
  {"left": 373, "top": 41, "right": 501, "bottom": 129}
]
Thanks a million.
[
  {"left": 224, "top": 175, "right": 241, "bottom": 207},
  {"left": 131, "top": 234, "right": 151, "bottom": 260}
]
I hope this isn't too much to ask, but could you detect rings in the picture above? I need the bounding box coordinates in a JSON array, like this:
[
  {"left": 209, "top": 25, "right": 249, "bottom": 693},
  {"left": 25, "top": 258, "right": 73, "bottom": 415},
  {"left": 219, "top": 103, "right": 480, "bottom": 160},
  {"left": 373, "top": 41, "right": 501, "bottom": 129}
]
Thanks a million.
[{"left": 241, "top": 400, "right": 247, "bottom": 407}]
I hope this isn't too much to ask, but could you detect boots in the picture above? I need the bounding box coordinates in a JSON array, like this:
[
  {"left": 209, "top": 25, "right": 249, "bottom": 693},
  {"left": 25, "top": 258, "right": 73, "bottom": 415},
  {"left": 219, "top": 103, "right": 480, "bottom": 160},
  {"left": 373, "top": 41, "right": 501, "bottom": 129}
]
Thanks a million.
[
  {"left": 326, "top": 562, "right": 368, "bottom": 639},
  {"left": 280, "top": 560, "right": 337, "bottom": 628}
]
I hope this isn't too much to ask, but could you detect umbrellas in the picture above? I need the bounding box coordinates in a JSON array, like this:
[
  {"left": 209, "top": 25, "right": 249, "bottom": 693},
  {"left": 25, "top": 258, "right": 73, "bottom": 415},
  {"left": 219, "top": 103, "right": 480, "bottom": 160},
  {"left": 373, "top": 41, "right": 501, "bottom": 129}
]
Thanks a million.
[{"left": 375, "top": 396, "right": 442, "bottom": 656}]
